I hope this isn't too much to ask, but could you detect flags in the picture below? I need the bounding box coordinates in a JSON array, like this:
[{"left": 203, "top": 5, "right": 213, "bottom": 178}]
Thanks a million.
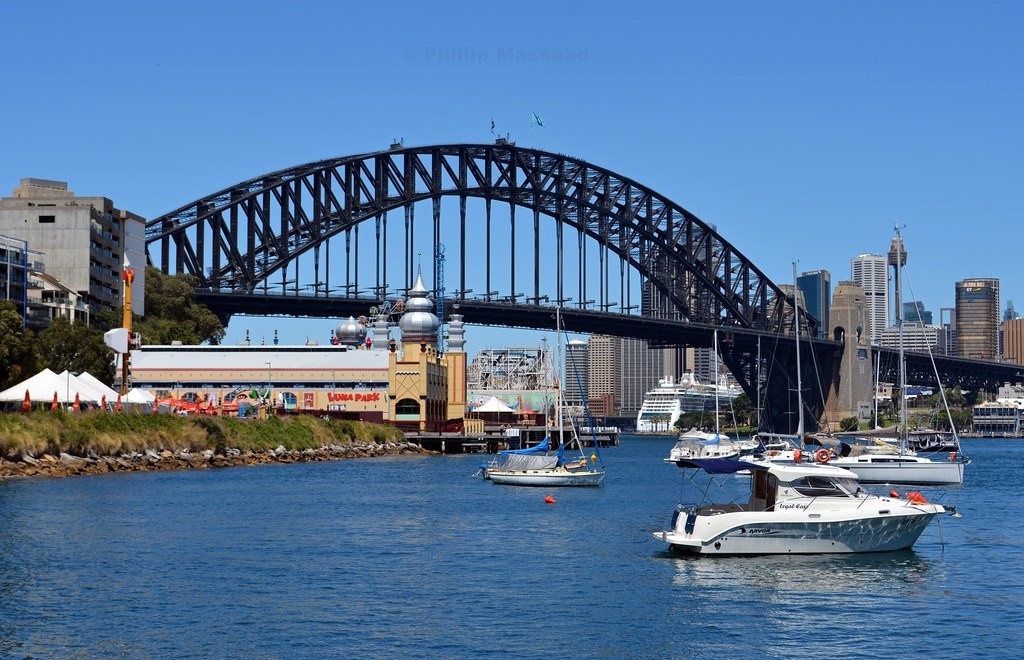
[
  {"left": 492, "top": 120, "right": 495, "bottom": 135},
  {"left": 532, "top": 112, "right": 545, "bottom": 128}
]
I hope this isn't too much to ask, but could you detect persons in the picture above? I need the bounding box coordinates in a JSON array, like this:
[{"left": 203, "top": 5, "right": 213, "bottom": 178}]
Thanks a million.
[
  {"left": 88, "top": 402, "right": 95, "bottom": 411},
  {"left": 67, "top": 402, "right": 74, "bottom": 412},
  {"left": 173, "top": 407, "right": 187, "bottom": 416}
]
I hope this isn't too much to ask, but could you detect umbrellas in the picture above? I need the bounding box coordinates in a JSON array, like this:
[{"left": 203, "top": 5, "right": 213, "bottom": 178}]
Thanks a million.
[
  {"left": 116, "top": 394, "right": 122, "bottom": 412},
  {"left": 51, "top": 391, "right": 58, "bottom": 412},
  {"left": 22, "top": 389, "right": 32, "bottom": 414},
  {"left": 209, "top": 400, "right": 212, "bottom": 416},
  {"left": 195, "top": 398, "right": 200, "bottom": 415},
  {"left": 72, "top": 392, "right": 80, "bottom": 412},
  {"left": 100, "top": 394, "right": 106, "bottom": 412},
  {"left": 152, "top": 397, "right": 158, "bottom": 412},
  {"left": 161, "top": 397, "right": 186, "bottom": 406}
]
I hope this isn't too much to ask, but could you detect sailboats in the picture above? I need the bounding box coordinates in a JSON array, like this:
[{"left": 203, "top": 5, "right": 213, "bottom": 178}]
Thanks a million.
[
  {"left": 662, "top": 223, "right": 972, "bottom": 485},
  {"left": 472, "top": 306, "right": 608, "bottom": 487}
]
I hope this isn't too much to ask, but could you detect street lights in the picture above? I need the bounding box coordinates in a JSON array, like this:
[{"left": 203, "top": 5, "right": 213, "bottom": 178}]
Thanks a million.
[{"left": 264, "top": 362, "right": 272, "bottom": 419}]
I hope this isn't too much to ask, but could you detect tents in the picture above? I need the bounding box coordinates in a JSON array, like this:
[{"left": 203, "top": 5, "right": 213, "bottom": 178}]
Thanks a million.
[
  {"left": 0, "top": 368, "right": 160, "bottom": 403},
  {"left": 471, "top": 396, "right": 516, "bottom": 423}
]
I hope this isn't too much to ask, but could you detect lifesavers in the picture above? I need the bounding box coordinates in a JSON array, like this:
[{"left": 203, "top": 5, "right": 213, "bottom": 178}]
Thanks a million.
[
  {"left": 940, "top": 441, "right": 947, "bottom": 448},
  {"left": 222, "top": 411, "right": 228, "bottom": 418},
  {"left": 794, "top": 450, "right": 802, "bottom": 462},
  {"left": 817, "top": 448, "right": 829, "bottom": 462}
]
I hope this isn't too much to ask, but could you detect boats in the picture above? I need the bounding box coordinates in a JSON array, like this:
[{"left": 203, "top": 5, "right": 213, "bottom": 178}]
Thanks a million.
[{"left": 654, "top": 463, "right": 964, "bottom": 555}]
